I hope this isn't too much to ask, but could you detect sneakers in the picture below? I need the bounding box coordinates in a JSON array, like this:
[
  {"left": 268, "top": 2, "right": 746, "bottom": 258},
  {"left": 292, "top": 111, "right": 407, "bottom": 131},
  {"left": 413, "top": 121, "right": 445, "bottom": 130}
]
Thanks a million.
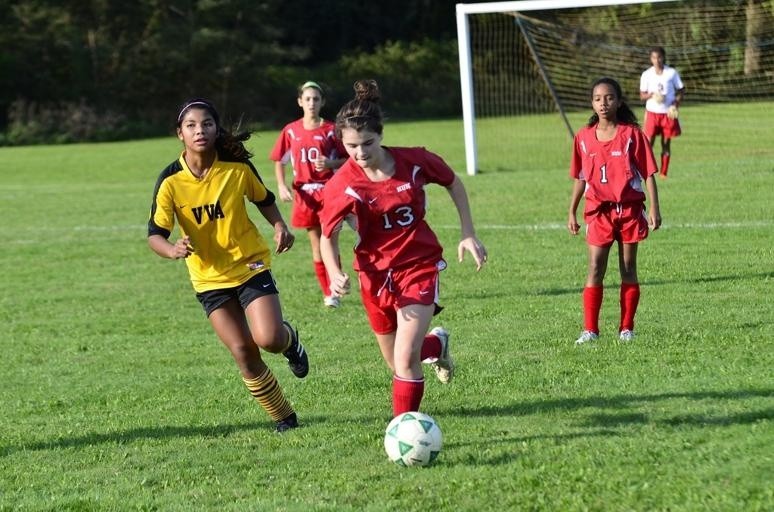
[
  {"left": 575, "top": 330, "right": 598, "bottom": 344},
  {"left": 282, "top": 321, "right": 309, "bottom": 377},
  {"left": 421, "top": 328, "right": 453, "bottom": 384},
  {"left": 277, "top": 414, "right": 297, "bottom": 433},
  {"left": 324, "top": 297, "right": 338, "bottom": 308},
  {"left": 620, "top": 330, "right": 634, "bottom": 341}
]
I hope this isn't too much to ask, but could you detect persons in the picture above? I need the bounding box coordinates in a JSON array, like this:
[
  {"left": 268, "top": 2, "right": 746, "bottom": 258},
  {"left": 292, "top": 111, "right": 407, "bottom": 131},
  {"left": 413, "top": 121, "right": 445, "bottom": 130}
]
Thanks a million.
[
  {"left": 566, "top": 78, "right": 664, "bottom": 345},
  {"left": 144, "top": 98, "right": 309, "bottom": 433},
  {"left": 317, "top": 78, "right": 487, "bottom": 420},
  {"left": 638, "top": 45, "right": 684, "bottom": 181},
  {"left": 269, "top": 80, "right": 357, "bottom": 308}
]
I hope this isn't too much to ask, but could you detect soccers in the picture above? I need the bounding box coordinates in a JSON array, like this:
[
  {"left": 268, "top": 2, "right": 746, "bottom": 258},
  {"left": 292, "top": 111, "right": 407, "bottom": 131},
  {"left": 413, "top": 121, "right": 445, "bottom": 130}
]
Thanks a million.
[{"left": 384, "top": 411, "right": 443, "bottom": 468}]
